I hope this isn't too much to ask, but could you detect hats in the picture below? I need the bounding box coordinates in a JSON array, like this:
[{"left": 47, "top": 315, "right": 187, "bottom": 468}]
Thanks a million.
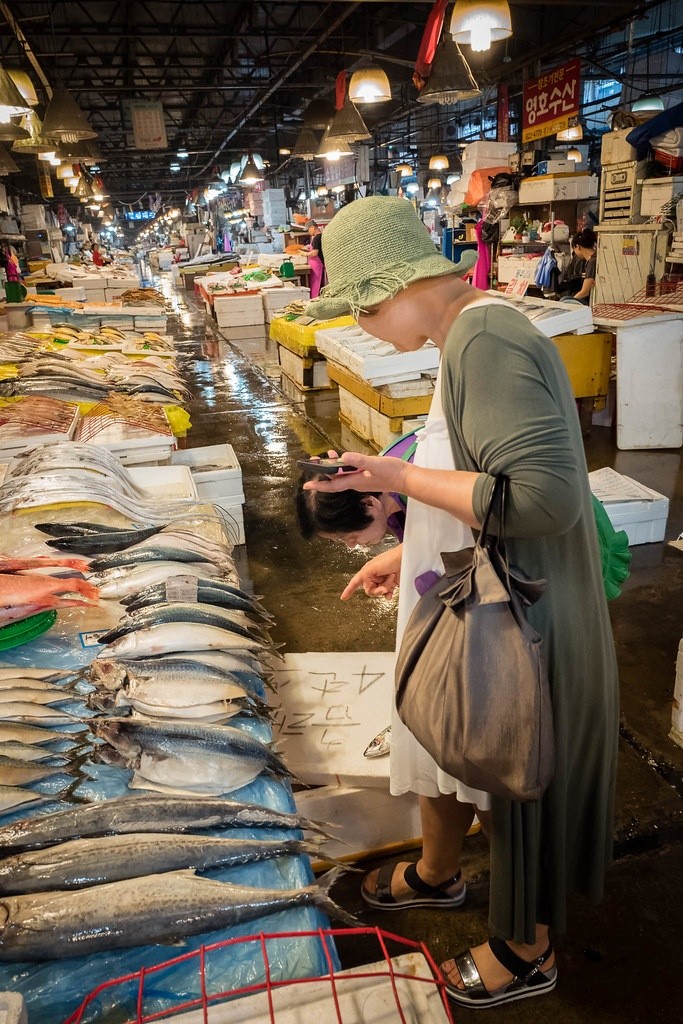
[
  {"left": 303, "top": 220, "right": 316, "bottom": 232},
  {"left": 285, "top": 195, "right": 479, "bottom": 319}
]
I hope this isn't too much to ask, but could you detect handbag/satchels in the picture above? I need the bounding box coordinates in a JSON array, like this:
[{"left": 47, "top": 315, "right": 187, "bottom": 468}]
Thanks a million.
[{"left": 391, "top": 473, "right": 553, "bottom": 802}]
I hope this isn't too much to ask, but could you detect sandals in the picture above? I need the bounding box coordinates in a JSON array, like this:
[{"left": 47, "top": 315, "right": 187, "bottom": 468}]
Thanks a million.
[
  {"left": 360, "top": 862, "right": 468, "bottom": 911},
  {"left": 439, "top": 936, "right": 557, "bottom": 1009}
]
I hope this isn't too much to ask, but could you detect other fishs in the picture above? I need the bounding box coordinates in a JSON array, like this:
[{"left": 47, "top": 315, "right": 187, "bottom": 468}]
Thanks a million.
[
  {"left": 1, "top": 519, "right": 368, "bottom": 959},
  {"left": 49, "top": 322, "right": 176, "bottom": 353},
  {"left": 0, "top": 352, "right": 197, "bottom": 406},
  {"left": 0, "top": 331, "right": 43, "bottom": 366}
]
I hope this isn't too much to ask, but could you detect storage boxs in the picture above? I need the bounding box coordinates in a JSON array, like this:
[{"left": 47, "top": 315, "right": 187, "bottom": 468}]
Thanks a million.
[
  {"left": 258, "top": 652, "right": 481, "bottom": 875},
  {"left": 148, "top": 127, "right": 683, "bottom": 548},
  {"left": 1, "top": 205, "right": 247, "bottom": 546}
]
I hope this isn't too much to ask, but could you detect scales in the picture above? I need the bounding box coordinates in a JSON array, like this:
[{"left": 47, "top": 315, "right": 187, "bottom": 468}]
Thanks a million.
[
  {"left": 279, "top": 258, "right": 295, "bottom": 277},
  {"left": 3, "top": 274, "right": 30, "bottom": 302}
]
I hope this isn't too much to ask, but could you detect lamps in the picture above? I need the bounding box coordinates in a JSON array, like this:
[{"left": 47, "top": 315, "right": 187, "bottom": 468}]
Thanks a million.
[
  {"left": 238, "top": 150, "right": 264, "bottom": 185},
  {"left": 556, "top": 122, "right": 583, "bottom": 141},
  {"left": 348, "top": 56, "right": 393, "bottom": 105},
  {"left": 0, "top": 30, "right": 115, "bottom": 226},
  {"left": 396, "top": 162, "right": 413, "bottom": 177},
  {"left": 290, "top": 85, "right": 372, "bottom": 161},
  {"left": 416, "top": 18, "right": 481, "bottom": 105},
  {"left": 446, "top": 175, "right": 460, "bottom": 185},
  {"left": 449, "top": 0, "right": 513, "bottom": 51},
  {"left": 567, "top": 147, "right": 583, "bottom": 163},
  {"left": 427, "top": 177, "right": 442, "bottom": 190},
  {"left": 429, "top": 146, "right": 449, "bottom": 170},
  {"left": 316, "top": 186, "right": 329, "bottom": 196},
  {"left": 407, "top": 181, "right": 419, "bottom": 194}
]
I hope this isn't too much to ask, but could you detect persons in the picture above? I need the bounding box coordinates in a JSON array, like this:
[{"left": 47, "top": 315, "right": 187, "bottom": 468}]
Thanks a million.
[
  {"left": 293, "top": 426, "right": 632, "bottom": 600},
  {"left": 91, "top": 243, "right": 112, "bottom": 266},
  {"left": 306, "top": 195, "right": 621, "bottom": 1008},
  {"left": 298, "top": 220, "right": 325, "bottom": 298},
  {"left": 571, "top": 228, "right": 597, "bottom": 298},
  {"left": 123, "top": 240, "right": 129, "bottom": 252}
]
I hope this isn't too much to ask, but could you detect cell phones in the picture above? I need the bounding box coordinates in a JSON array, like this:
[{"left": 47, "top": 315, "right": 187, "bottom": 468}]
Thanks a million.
[{"left": 297, "top": 458, "right": 359, "bottom": 474}]
